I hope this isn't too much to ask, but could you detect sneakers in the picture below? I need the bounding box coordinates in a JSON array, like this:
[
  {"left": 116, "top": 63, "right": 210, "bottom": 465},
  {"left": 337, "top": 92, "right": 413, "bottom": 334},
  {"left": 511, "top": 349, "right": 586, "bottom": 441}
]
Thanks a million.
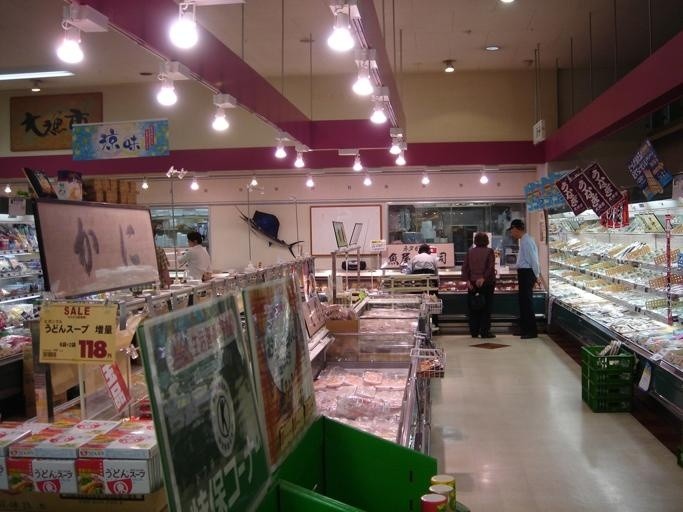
[
  {"left": 513, "top": 330, "right": 521, "bottom": 335},
  {"left": 472, "top": 335, "right": 477, "bottom": 337},
  {"left": 481, "top": 335, "right": 495, "bottom": 338},
  {"left": 521, "top": 335, "right": 537, "bottom": 338}
]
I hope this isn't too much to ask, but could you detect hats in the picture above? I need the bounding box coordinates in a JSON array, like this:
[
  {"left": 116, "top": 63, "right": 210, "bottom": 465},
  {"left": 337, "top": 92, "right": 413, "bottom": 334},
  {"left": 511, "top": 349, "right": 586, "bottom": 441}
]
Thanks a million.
[{"left": 507, "top": 219, "right": 523, "bottom": 231}]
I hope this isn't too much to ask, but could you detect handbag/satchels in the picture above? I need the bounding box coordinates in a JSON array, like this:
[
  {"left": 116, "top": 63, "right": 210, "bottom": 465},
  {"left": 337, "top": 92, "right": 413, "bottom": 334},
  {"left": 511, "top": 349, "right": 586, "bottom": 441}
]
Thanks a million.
[
  {"left": 482, "top": 277, "right": 495, "bottom": 298},
  {"left": 468, "top": 284, "right": 485, "bottom": 310}
]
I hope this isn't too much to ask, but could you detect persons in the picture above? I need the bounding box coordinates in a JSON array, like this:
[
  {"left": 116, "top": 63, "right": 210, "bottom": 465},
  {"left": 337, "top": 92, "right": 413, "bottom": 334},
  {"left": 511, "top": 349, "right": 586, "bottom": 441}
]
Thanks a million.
[
  {"left": 506, "top": 219, "right": 541, "bottom": 338},
  {"left": 461, "top": 233, "right": 496, "bottom": 338},
  {"left": 129, "top": 244, "right": 171, "bottom": 298},
  {"left": 407, "top": 245, "right": 440, "bottom": 327},
  {"left": 178, "top": 232, "right": 211, "bottom": 283}
]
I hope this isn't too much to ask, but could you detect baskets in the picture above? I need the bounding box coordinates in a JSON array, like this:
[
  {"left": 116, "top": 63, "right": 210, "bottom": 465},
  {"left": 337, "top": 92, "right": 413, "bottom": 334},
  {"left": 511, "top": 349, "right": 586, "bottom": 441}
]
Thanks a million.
[
  {"left": 581, "top": 374, "right": 633, "bottom": 412},
  {"left": 410, "top": 348, "right": 446, "bottom": 379},
  {"left": 425, "top": 298, "right": 442, "bottom": 315},
  {"left": 580, "top": 346, "right": 634, "bottom": 385}
]
